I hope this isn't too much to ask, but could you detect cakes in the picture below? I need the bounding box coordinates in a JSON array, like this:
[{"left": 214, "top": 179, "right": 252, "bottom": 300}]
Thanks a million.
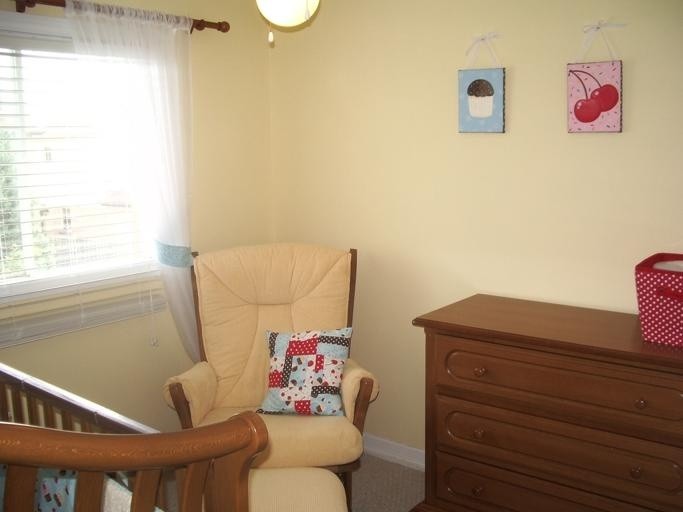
[{"left": 468, "top": 78, "right": 494, "bottom": 118}]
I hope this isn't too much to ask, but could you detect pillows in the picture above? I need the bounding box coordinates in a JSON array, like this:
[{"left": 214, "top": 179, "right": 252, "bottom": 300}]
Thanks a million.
[{"left": 256, "top": 324, "right": 354, "bottom": 418}]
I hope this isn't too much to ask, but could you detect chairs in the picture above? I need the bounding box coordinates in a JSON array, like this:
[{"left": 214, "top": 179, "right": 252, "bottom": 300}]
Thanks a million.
[
  {"left": 0, "top": 358, "right": 272, "bottom": 512},
  {"left": 164, "top": 242, "right": 382, "bottom": 512}
]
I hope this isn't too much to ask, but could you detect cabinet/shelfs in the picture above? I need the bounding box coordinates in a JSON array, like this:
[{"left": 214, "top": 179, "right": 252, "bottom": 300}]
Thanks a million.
[{"left": 410, "top": 294, "right": 682, "bottom": 512}]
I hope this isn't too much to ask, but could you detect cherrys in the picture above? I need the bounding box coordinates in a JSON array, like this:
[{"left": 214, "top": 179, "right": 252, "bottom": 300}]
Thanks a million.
[{"left": 569, "top": 69, "right": 619, "bottom": 123}]
